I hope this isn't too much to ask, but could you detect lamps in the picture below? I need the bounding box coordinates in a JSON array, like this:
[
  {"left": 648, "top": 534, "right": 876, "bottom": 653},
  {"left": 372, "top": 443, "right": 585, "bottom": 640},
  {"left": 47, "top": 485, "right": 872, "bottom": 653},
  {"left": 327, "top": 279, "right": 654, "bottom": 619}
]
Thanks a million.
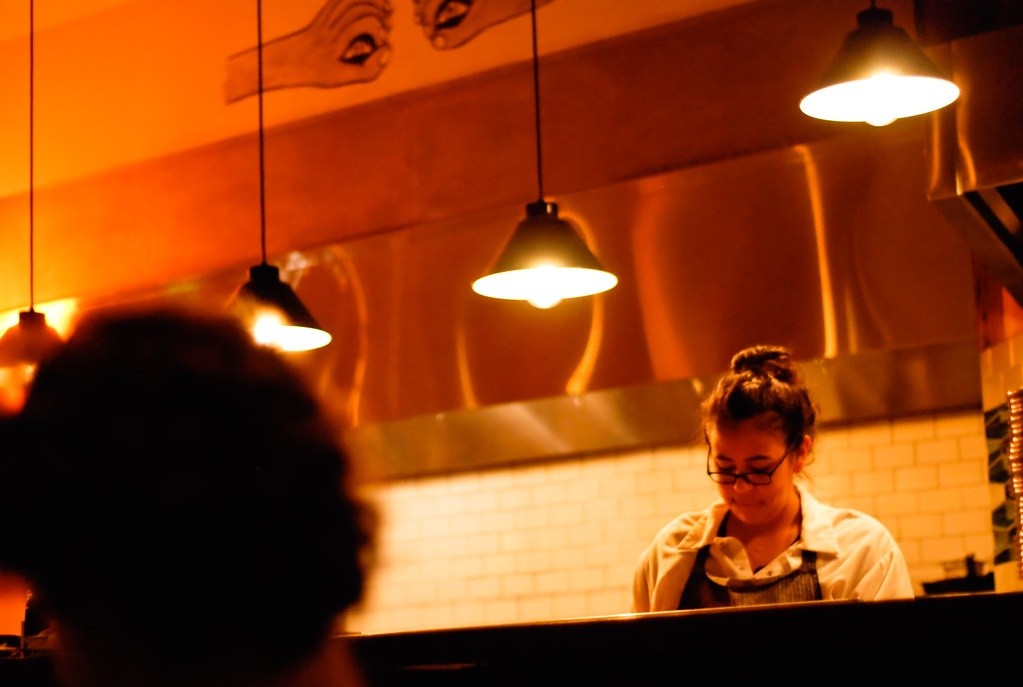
[
  {"left": 0, "top": 0, "right": 67, "bottom": 370},
  {"left": 800, "top": 0, "right": 962, "bottom": 127},
  {"left": 470, "top": 0, "right": 619, "bottom": 311},
  {"left": 222, "top": 1, "right": 333, "bottom": 350}
]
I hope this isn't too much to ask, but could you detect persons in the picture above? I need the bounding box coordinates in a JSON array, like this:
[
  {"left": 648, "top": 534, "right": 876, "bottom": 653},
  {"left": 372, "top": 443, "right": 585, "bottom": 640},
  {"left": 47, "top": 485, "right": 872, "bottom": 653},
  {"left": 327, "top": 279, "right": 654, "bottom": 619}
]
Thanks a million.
[
  {"left": 0, "top": 297, "right": 386, "bottom": 687},
  {"left": 635, "top": 345, "right": 914, "bottom": 616}
]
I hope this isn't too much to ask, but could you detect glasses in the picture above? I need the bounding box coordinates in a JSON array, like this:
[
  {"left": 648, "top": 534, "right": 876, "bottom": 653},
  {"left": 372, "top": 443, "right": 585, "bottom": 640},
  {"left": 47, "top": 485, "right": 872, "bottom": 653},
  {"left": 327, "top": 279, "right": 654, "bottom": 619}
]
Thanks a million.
[{"left": 707, "top": 442, "right": 795, "bottom": 486}]
